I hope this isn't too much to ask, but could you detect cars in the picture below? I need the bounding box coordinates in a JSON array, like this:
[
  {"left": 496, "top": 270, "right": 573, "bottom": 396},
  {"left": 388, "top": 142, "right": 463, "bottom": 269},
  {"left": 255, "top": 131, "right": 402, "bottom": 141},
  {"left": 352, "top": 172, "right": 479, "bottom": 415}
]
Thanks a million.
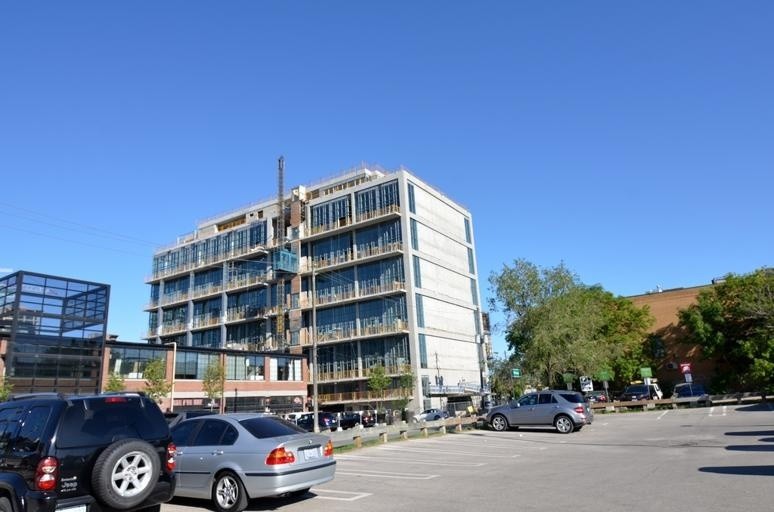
[
  {"left": 412, "top": 408, "right": 449, "bottom": 423},
  {"left": 486, "top": 389, "right": 593, "bottom": 434},
  {"left": 170, "top": 412, "right": 335, "bottom": 511},
  {"left": 297, "top": 411, "right": 375, "bottom": 431},
  {"left": 585, "top": 391, "right": 614, "bottom": 403}
]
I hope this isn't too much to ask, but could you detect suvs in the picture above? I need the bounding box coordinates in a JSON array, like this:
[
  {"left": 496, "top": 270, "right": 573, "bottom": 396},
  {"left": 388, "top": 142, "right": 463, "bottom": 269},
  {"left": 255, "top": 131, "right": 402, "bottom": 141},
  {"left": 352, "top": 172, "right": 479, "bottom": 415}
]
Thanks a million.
[
  {"left": 621, "top": 385, "right": 659, "bottom": 408},
  {"left": 0, "top": 391, "right": 177, "bottom": 511}
]
